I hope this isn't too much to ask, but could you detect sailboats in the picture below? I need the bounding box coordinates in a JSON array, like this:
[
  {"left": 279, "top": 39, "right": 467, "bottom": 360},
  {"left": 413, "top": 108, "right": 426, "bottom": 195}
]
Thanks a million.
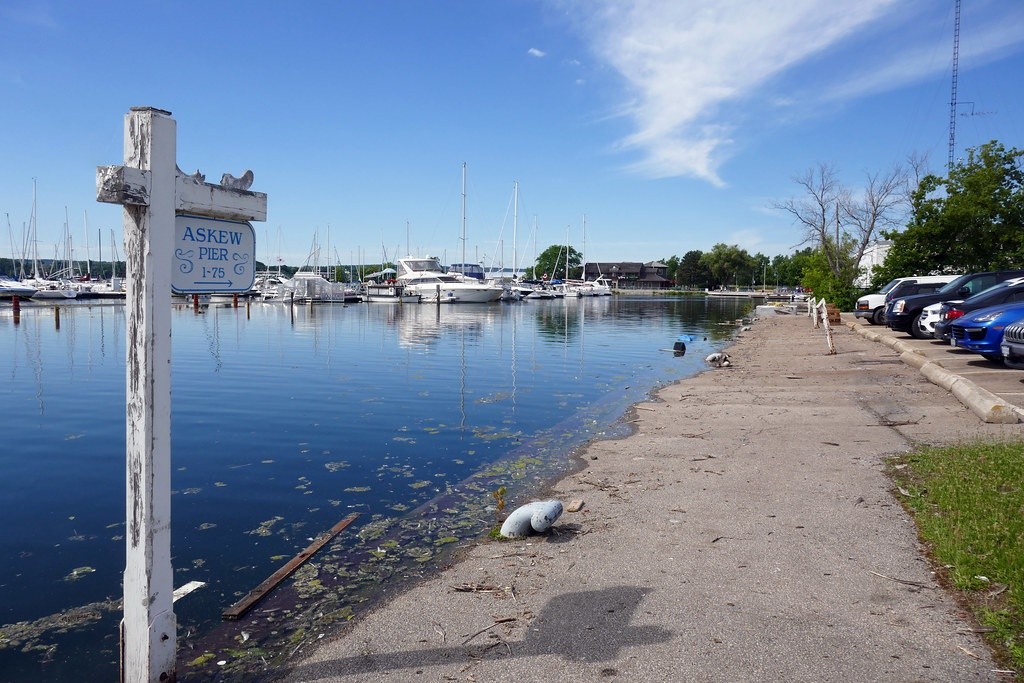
[
  {"left": 441, "top": 161, "right": 614, "bottom": 299},
  {"left": 0, "top": 177, "right": 398, "bottom": 303}
]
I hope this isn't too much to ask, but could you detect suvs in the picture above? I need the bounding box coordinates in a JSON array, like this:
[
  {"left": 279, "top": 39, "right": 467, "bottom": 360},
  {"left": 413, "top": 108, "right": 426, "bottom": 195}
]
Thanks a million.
[{"left": 885, "top": 282, "right": 947, "bottom": 311}]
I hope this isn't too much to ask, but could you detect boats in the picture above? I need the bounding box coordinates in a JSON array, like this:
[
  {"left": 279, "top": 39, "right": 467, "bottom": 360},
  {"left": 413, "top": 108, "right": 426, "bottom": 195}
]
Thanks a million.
[
  {"left": 361, "top": 283, "right": 421, "bottom": 303},
  {"left": 395, "top": 254, "right": 503, "bottom": 303}
]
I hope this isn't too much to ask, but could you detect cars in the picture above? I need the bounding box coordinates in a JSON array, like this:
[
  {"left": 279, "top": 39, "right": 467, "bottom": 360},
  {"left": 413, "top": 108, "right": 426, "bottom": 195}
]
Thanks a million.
[
  {"left": 933, "top": 277, "right": 1024, "bottom": 345},
  {"left": 947, "top": 301, "right": 1024, "bottom": 365},
  {"left": 917, "top": 277, "right": 1024, "bottom": 340},
  {"left": 884, "top": 270, "right": 1024, "bottom": 339},
  {"left": 1000, "top": 318, "right": 1024, "bottom": 369}
]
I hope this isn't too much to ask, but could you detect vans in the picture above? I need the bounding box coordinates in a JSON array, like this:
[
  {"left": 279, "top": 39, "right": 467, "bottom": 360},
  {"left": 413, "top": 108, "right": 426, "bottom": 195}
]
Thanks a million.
[{"left": 855, "top": 275, "right": 965, "bottom": 325}]
